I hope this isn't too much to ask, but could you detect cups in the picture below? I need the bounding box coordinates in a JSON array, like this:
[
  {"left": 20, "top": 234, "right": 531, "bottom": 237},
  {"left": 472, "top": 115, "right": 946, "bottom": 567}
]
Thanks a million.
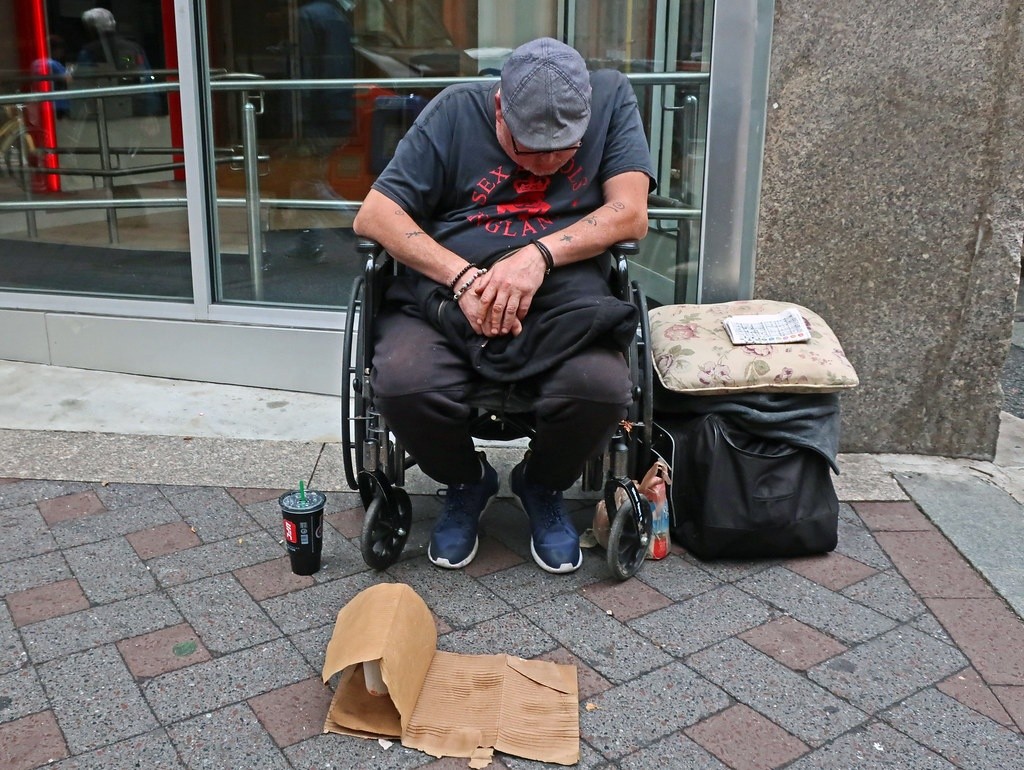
[{"left": 279, "top": 489, "right": 326, "bottom": 576}]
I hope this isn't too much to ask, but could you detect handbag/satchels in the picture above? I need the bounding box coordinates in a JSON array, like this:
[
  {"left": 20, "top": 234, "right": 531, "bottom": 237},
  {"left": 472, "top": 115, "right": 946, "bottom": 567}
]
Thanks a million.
[{"left": 646, "top": 377, "right": 842, "bottom": 560}]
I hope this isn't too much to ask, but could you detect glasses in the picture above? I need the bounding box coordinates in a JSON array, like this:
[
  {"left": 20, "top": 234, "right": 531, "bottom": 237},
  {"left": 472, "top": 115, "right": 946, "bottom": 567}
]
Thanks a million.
[{"left": 510, "top": 134, "right": 584, "bottom": 156}]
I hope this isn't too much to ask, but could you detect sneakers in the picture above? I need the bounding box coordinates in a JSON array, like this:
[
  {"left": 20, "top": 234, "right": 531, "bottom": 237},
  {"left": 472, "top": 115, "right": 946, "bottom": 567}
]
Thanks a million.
[
  {"left": 427, "top": 451, "right": 501, "bottom": 569},
  {"left": 508, "top": 462, "right": 583, "bottom": 572}
]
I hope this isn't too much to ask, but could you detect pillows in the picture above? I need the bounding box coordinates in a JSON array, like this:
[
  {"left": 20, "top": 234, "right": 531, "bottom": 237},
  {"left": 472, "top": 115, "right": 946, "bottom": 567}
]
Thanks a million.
[{"left": 647, "top": 298, "right": 860, "bottom": 397}]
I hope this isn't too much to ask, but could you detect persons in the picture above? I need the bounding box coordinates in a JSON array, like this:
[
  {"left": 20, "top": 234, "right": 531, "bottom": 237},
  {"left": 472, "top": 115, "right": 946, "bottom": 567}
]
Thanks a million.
[
  {"left": 30, "top": 0, "right": 357, "bottom": 136},
  {"left": 353, "top": 38, "right": 657, "bottom": 573}
]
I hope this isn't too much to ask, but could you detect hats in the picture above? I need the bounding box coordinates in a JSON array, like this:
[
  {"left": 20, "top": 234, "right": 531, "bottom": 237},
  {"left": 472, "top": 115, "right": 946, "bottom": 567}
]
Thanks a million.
[{"left": 500, "top": 37, "right": 593, "bottom": 151}]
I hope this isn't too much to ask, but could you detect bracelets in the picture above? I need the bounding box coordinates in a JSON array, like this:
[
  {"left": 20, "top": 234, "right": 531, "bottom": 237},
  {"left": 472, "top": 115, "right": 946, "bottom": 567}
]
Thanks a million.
[
  {"left": 528, "top": 238, "right": 554, "bottom": 280},
  {"left": 453, "top": 267, "right": 488, "bottom": 303},
  {"left": 448, "top": 262, "right": 477, "bottom": 290}
]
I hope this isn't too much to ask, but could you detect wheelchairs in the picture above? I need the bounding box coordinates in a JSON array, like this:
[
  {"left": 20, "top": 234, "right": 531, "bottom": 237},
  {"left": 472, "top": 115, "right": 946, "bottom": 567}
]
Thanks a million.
[{"left": 342, "top": 235, "right": 654, "bottom": 581}]
